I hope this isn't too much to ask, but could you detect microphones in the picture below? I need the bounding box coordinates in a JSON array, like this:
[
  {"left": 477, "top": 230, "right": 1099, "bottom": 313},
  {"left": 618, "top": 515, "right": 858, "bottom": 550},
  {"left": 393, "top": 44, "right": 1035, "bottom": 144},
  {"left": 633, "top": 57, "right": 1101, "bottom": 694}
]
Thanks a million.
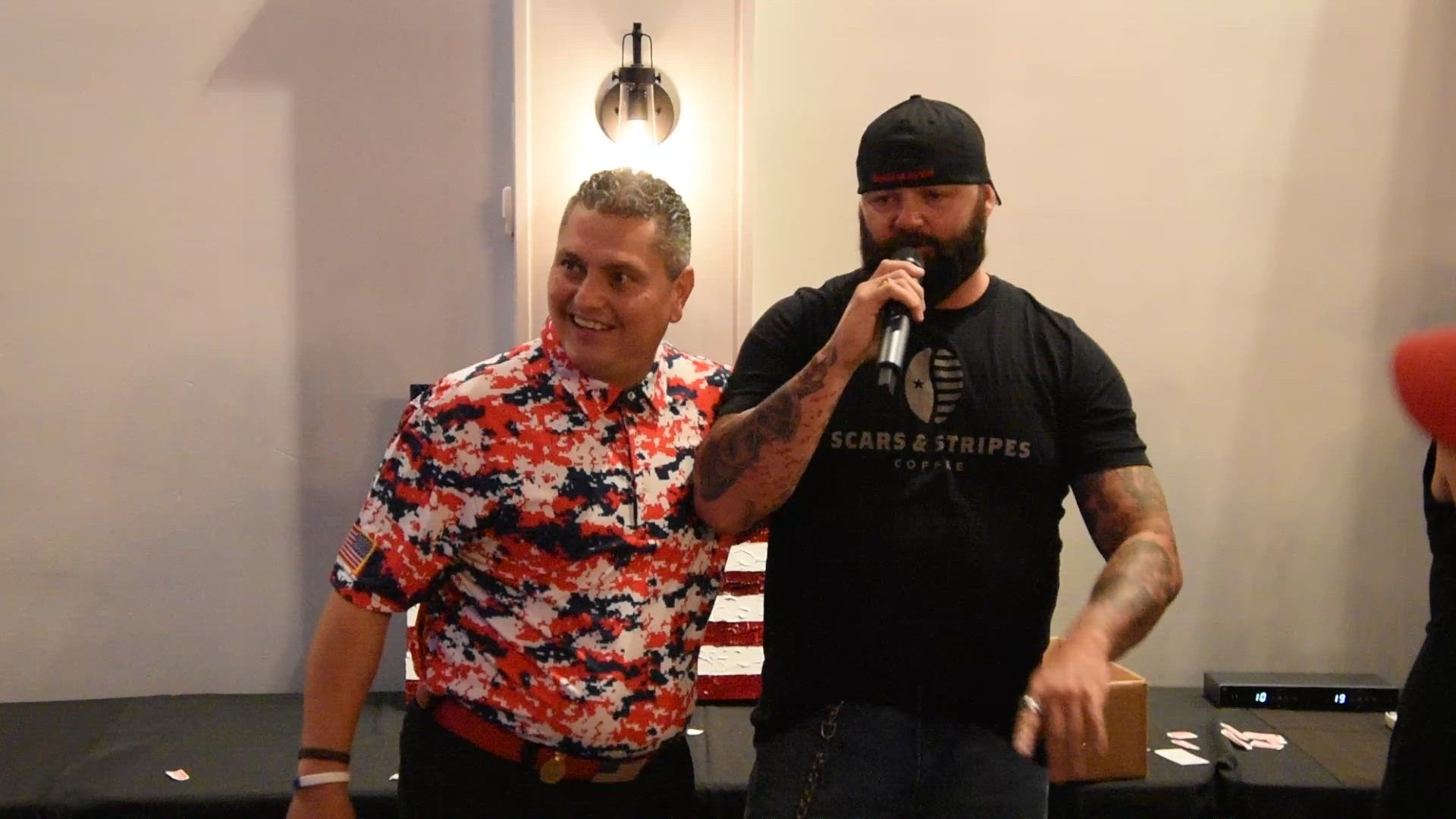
[{"left": 874, "top": 246, "right": 925, "bottom": 396}]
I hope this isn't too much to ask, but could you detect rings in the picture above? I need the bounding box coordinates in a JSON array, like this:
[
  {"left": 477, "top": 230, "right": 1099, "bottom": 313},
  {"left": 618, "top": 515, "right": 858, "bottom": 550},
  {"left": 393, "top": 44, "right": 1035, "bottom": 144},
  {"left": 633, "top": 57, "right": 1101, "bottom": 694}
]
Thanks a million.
[{"left": 1020, "top": 695, "right": 1042, "bottom": 716}]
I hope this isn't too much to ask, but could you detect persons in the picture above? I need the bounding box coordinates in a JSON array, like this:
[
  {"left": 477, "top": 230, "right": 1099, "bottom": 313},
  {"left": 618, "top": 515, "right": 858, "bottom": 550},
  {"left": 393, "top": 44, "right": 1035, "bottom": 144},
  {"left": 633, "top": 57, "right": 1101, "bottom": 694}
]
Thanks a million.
[
  {"left": 270, "top": 167, "right": 735, "bottom": 819},
  {"left": 1376, "top": 436, "right": 1456, "bottom": 819},
  {"left": 693, "top": 93, "right": 1183, "bottom": 819}
]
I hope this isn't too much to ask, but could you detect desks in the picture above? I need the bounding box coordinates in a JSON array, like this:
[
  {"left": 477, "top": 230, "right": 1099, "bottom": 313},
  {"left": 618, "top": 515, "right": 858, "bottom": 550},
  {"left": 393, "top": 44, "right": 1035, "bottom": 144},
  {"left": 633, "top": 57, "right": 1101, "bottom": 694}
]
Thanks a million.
[{"left": 0, "top": 683, "right": 1399, "bottom": 819}]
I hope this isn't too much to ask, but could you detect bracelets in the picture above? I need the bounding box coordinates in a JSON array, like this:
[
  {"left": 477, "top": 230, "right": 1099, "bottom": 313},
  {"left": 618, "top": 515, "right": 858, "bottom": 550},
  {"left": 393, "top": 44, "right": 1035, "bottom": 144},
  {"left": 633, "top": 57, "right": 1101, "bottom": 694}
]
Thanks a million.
[
  {"left": 293, "top": 772, "right": 351, "bottom": 788},
  {"left": 298, "top": 747, "right": 350, "bottom": 764}
]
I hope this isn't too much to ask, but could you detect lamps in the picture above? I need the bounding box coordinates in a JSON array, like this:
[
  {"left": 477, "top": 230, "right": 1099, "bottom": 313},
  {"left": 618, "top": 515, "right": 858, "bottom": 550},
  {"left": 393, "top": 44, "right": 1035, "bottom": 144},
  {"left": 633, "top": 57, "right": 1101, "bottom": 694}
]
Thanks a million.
[{"left": 594, "top": 22, "right": 681, "bottom": 150}]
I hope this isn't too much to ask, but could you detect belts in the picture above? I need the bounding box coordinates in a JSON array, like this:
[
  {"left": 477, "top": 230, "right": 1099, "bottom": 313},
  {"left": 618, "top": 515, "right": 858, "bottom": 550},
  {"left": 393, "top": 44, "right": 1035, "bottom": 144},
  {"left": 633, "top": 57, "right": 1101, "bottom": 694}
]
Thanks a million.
[{"left": 428, "top": 697, "right": 671, "bottom": 785}]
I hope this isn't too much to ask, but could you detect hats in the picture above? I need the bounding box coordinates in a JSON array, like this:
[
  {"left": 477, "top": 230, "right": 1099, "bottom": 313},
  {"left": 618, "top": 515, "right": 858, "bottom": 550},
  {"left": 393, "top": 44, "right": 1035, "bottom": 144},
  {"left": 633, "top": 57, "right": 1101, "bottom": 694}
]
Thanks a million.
[
  {"left": 855, "top": 92, "right": 1004, "bottom": 206},
  {"left": 1393, "top": 325, "right": 1456, "bottom": 444}
]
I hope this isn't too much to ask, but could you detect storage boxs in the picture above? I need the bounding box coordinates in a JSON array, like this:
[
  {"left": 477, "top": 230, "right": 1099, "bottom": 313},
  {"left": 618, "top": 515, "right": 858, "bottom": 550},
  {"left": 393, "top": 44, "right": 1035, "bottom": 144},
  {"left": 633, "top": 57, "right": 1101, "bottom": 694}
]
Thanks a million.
[{"left": 1042, "top": 635, "right": 1147, "bottom": 781}]
